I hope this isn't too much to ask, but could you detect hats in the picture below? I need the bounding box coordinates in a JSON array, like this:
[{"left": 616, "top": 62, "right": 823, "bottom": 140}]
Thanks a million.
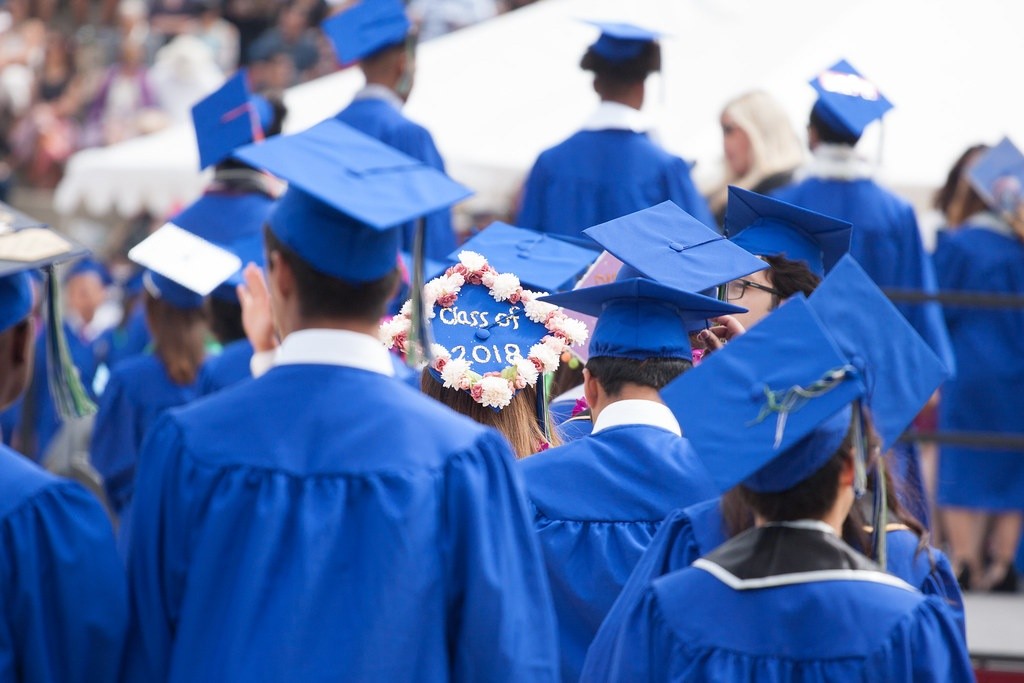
[
  {"left": 580, "top": 199, "right": 771, "bottom": 292},
  {"left": 71, "top": 257, "right": 114, "bottom": 290},
  {"left": 584, "top": 17, "right": 662, "bottom": 67},
  {"left": 0, "top": 202, "right": 99, "bottom": 419},
  {"left": 810, "top": 58, "right": 894, "bottom": 137},
  {"left": 231, "top": 117, "right": 476, "bottom": 367},
  {"left": 535, "top": 276, "right": 750, "bottom": 363},
  {"left": 964, "top": 136, "right": 1024, "bottom": 220},
  {"left": 171, "top": 190, "right": 278, "bottom": 285},
  {"left": 721, "top": 184, "right": 853, "bottom": 279},
  {"left": 447, "top": 220, "right": 599, "bottom": 291},
  {"left": 323, "top": 0, "right": 415, "bottom": 96},
  {"left": 122, "top": 266, "right": 208, "bottom": 310},
  {"left": 805, "top": 252, "right": 951, "bottom": 571},
  {"left": 379, "top": 249, "right": 590, "bottom": 446},
  {"left": 657, "top": 292, "right": 888, "bottom": 572},
  {"left": 190, "top": 70, "right": 274, "bottom": 172}
]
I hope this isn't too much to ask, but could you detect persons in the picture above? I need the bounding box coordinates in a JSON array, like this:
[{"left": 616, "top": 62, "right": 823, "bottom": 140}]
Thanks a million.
[
  {"left": 411, "top": 16, "right": 1023, "bottom": 625},
  {"left": 0, "top": 195, "right": 119, "bottom": 683},
  {"left": 0, "top": 0, "right": 522, "bottom": 537},
  {"left": 605, "top": 294, "right": 974, "bottom": 683},
  {"left": 112, "top": 120, "right": 564, "bottom": 683}
]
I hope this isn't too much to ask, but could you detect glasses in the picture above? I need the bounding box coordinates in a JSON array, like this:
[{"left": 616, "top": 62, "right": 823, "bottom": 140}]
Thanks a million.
[{"left": 725, "top": 278, "right": 787, "bottom": 300}]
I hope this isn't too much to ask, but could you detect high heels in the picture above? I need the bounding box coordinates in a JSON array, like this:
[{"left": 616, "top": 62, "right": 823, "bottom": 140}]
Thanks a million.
[
  {"left": 956, "top": 567, "right": 973, "bottom": 593},
  {"left": 991, "top": 564, "right": 1017, "bottom": 594}
]
